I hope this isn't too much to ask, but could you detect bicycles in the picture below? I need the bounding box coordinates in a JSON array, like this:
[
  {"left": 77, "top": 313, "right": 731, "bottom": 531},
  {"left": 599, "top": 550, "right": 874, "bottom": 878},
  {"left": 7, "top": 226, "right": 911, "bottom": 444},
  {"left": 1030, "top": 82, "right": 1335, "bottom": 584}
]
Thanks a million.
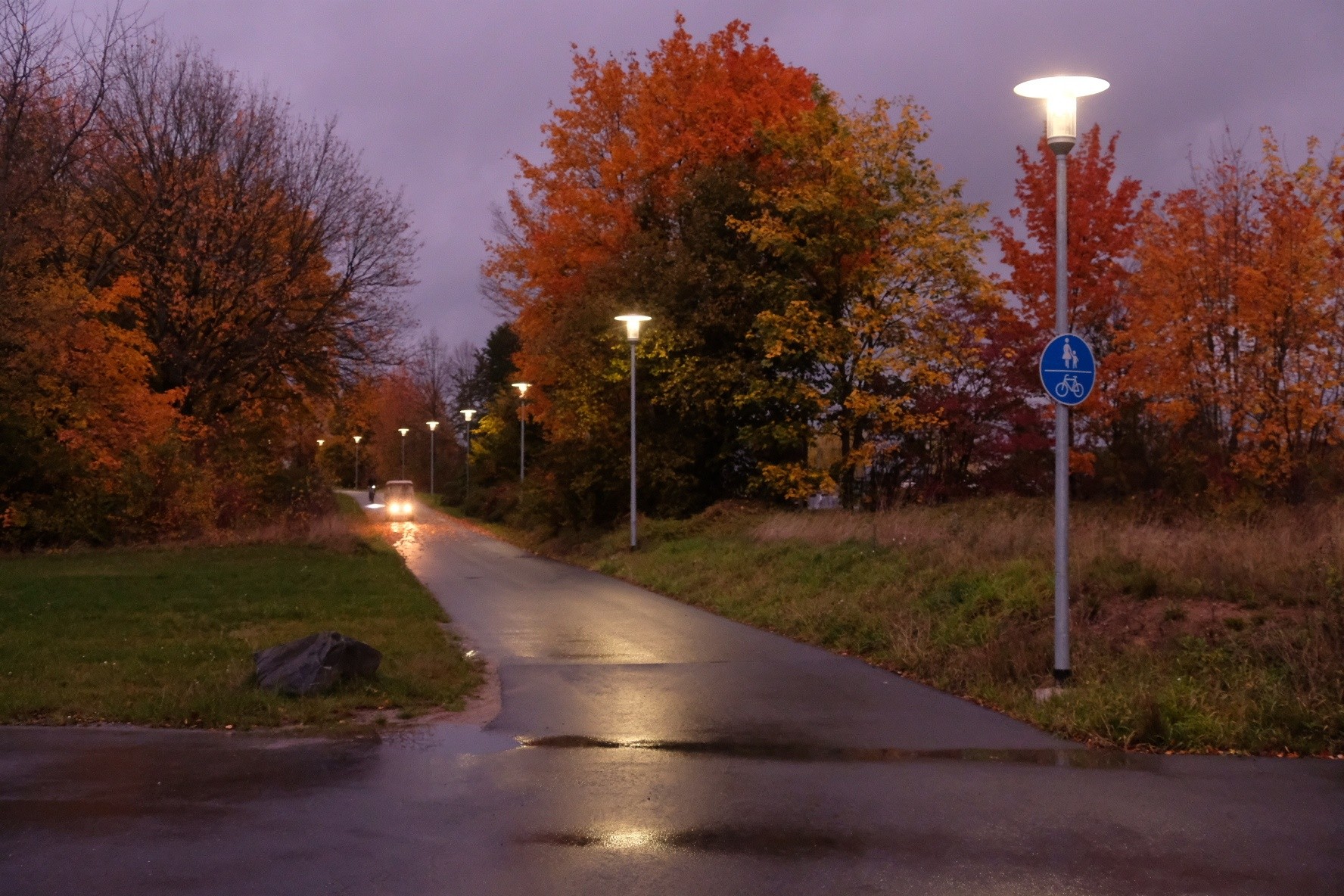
[{"left": 368, "top": 484, "right": 377, "bottom": 503}]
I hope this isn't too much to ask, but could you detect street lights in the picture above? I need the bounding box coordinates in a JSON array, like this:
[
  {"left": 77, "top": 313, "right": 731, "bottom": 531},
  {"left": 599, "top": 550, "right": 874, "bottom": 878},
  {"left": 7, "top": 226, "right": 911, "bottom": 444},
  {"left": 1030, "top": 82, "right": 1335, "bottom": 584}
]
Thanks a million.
[
  {"left": 1014, "top": 75, "right": 1111, "bottom": 682},
  {"left": 614, "top": 312, "right": 652, "bottom": 539},
  {"left": 353, "top": 436, "right": 362, "bottom": 492},
  {"left": 398, "top": 428, "right": 410, "bottom": 479},
  {"left": 427, "top": 420, "right": 439, "bottom": 500},
  {"left": 511, "top": 380, "right": 532, "bottom": 504},
  {"left": 316, "top": 439, "right": 327, "bottom": 475},
  {"left": 460, "top": 409, "right": 478, "bottom": 513}
]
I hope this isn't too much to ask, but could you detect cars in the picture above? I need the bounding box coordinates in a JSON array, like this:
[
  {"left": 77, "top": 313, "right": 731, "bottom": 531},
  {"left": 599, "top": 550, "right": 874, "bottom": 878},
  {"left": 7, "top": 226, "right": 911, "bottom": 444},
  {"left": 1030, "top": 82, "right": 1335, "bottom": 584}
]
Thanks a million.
[{"left": 384, "top": 480, "right": 416, "bottom": 521}]
